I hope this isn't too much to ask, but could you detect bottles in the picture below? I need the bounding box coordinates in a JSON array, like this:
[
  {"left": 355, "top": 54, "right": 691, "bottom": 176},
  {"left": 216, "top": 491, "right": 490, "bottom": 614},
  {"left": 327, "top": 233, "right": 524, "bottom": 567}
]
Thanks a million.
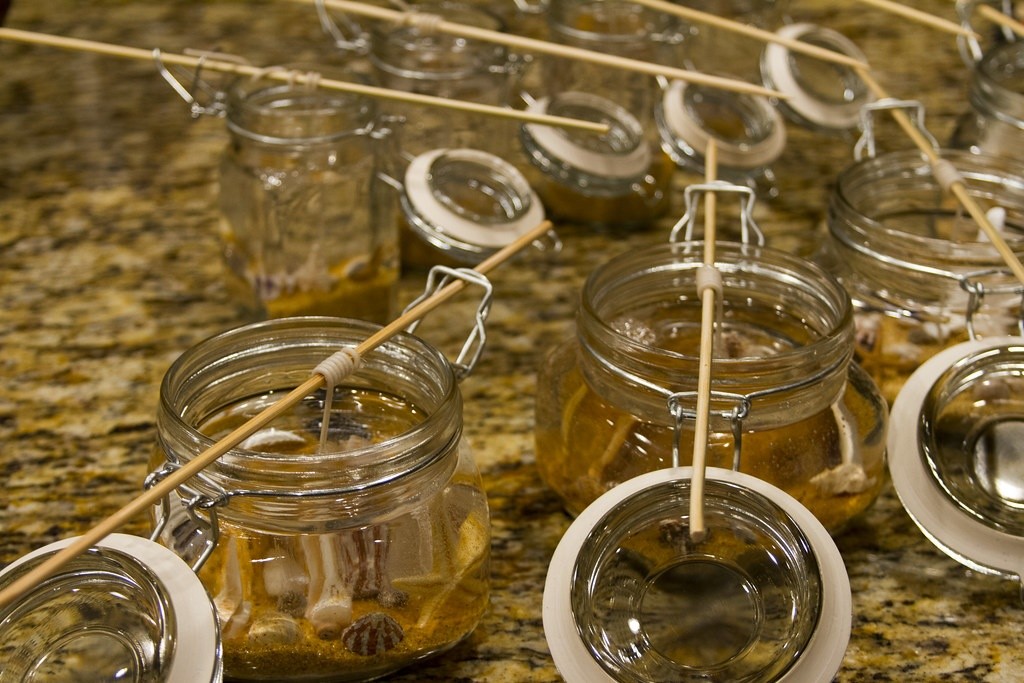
[{"left": 155, "top": 1, "right": 1024, "bottom": 682}]
